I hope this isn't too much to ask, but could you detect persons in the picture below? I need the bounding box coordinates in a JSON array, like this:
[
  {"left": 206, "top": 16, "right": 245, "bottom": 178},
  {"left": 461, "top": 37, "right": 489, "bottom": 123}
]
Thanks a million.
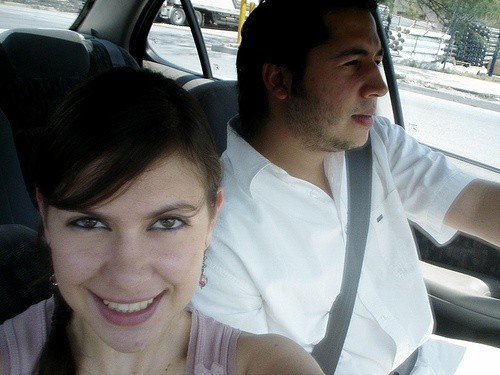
[
  {"left": 0, "top": 66, "right": 327, "bottom": 375},
  {"left": 187, "top": 0, "right": 500, "bottom": 375}
]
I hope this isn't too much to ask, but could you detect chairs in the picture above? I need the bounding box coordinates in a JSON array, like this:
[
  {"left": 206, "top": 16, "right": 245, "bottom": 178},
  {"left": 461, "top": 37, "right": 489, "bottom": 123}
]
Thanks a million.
[{"left": 0, "top": 28, "right": 239, "bottom": 324}]
